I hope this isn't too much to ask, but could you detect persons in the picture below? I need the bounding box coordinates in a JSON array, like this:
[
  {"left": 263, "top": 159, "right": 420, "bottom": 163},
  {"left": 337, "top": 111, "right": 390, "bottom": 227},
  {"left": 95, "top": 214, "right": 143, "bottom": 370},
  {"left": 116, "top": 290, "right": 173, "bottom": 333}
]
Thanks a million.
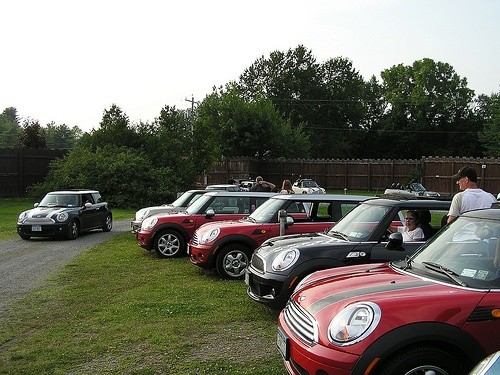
[
  {"left": 280, "top": 180, "right": 294, "bottom": 196},
  {"left": 445, "top": 166, "right": 498, "bottom": 224},
  {"left": 227, "top": 178, "right": 246, "bottom": 214},
  {"left": 249, "top": 176, "right": 276, "bottom": 193},
  {"left": 399, "top": 211, "right": 426, "bottom": 243}
]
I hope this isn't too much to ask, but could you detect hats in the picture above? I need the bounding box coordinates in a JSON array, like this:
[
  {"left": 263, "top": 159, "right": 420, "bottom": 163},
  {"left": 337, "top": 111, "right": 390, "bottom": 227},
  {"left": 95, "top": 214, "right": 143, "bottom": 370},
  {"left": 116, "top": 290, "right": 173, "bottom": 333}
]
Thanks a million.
[{"left": 451, "top": 166, "right": 476, "bottom": 178}]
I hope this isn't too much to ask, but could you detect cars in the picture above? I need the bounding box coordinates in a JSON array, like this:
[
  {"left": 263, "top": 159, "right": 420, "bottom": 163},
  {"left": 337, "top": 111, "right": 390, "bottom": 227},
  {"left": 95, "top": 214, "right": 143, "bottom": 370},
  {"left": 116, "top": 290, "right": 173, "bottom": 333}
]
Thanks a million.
[
  {"left": 275, "top": 202, "right": 500, "bottom": 375},
  {"left": 291, "top": 180, "right": 326, "bottom": 194},
  {"left": 187, "top": 193, "right": 407, "bottom": 280},
  {"left": 130, "top": 184, "right": 310, "bottom": 258},
  {"left": 383, "top": 182, "right": 439, "bottom": 200},
  {"left": 16, "top": 188, "right": 112, "bottom": 241},
  {"left": 244, "top": 198, "right": 453, "bottom": 310}
]
